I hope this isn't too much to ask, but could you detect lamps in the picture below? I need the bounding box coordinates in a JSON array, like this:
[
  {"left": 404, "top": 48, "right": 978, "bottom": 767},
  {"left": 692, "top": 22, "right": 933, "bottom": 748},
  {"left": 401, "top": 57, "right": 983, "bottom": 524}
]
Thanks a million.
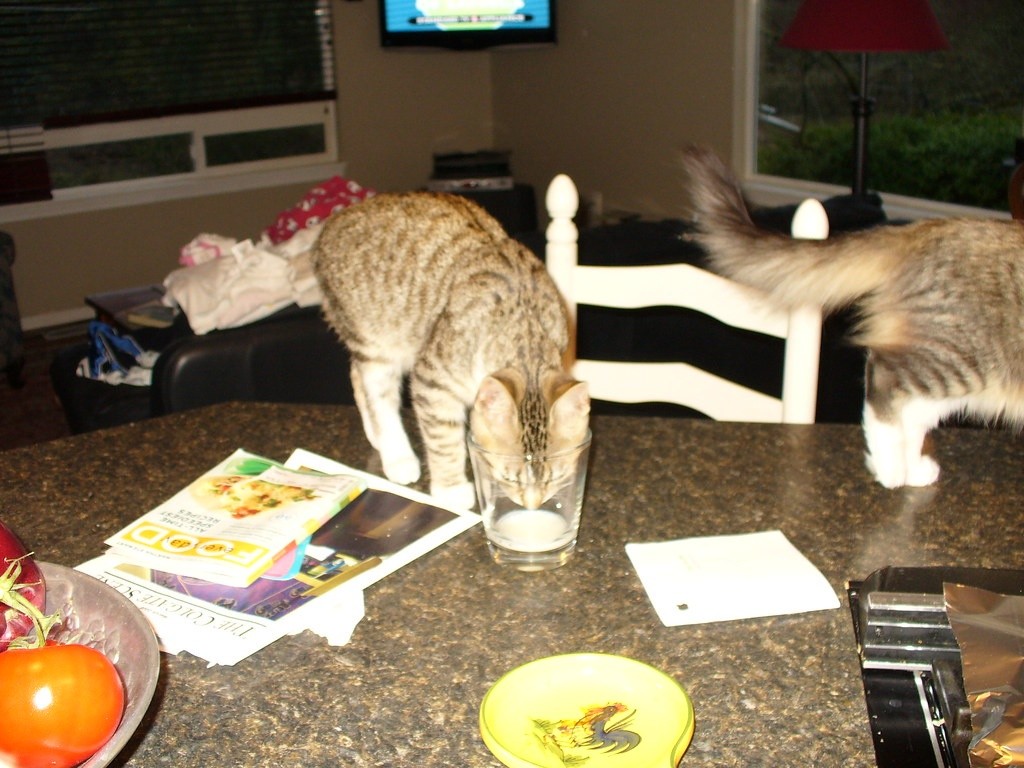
[{"left": 779, "top": 0, "right": 953, "bottom": 228}]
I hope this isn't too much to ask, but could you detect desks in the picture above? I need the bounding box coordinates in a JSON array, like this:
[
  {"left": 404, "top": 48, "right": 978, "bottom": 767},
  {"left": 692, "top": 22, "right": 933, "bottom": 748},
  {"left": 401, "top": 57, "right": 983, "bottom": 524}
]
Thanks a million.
[{"left": 0, "top": 400, "right": 1024, "bottom": 766}]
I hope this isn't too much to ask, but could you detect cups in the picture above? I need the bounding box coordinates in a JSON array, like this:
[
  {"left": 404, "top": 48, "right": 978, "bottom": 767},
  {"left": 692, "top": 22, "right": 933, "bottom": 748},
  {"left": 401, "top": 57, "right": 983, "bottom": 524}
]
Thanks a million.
[{"left": 463, "top": 428, "right": 593, "bottom": 569}]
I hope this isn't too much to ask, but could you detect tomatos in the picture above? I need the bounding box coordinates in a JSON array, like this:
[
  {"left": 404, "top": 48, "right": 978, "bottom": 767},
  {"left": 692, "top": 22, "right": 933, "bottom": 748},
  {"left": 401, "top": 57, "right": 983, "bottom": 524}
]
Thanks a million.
[{"left": 0, "top": 552, "right": 126, "bottom": 768}]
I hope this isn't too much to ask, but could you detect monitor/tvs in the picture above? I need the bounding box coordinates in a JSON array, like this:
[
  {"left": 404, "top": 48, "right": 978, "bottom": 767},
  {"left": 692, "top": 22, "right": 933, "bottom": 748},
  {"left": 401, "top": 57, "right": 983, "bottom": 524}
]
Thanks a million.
[{"left": 376, "top": 0, "right": 559, "bottom": 49}]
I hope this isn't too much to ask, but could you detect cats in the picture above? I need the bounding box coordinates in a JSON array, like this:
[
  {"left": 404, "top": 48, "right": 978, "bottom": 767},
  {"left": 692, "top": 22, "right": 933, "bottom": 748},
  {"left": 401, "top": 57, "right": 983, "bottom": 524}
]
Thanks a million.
[
  {"left": 677, "top": 138, "right": 1024, "bottom": 492},
  {"left": 260, "top": 190, "right": 593, "bottom": 521}
]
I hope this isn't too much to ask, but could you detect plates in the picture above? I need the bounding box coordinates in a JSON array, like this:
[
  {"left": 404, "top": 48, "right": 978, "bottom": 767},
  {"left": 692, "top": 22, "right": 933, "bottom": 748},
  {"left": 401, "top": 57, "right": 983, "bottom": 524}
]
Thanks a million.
[
  {"left": 478, "top": 650, "right": 697, "bottom": 768},
  {"left": 0, "top": 562, "right": 162, "bottom": 768}
]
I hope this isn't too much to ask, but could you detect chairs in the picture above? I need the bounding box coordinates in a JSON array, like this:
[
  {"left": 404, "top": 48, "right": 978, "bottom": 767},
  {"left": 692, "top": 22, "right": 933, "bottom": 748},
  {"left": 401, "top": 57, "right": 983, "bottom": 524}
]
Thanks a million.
[{"left": 539, "top": 175, "right": 828, "bottom": 425}]
